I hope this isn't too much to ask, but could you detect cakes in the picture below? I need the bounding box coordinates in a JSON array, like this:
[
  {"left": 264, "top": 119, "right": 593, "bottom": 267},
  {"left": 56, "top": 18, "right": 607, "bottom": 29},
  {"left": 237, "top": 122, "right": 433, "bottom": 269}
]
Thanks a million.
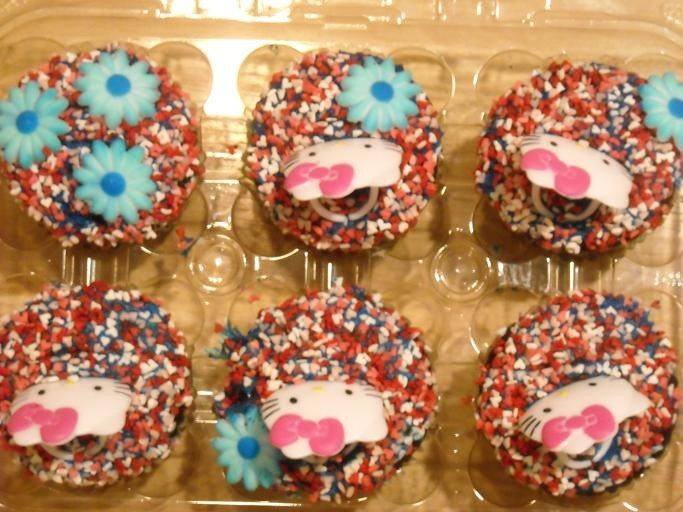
[
  {"left": 0, "top": 282, "right": 193, "bottom": 487},
  {"left": 474, "top": 60, "right": 683, "bottom": 257},
  {"left": 233, "top": 45, "right": 442, "bottom": 252},
  {"left": 0, "top": 49, "right": 201, "bottom": 251},
  {"left": 472, "top": 286, "right": 681, "bottom": 498},
  {"left": 209, "top": 285, "right": 435, "bottom": 507}
]
